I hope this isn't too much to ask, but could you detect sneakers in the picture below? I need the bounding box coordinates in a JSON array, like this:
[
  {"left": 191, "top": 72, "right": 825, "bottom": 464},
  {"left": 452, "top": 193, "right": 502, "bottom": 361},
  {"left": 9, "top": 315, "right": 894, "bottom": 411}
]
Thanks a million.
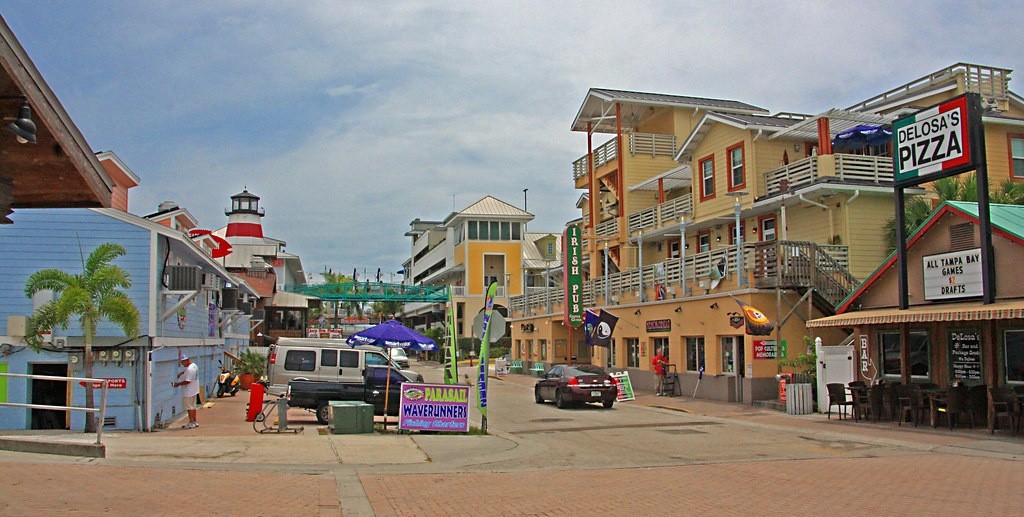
[
  {"left": 182, "top": 423, "right": 195, "bottom": 428},
  {"left": 194, "top": 422, "right": 199, "bottom": 426}
]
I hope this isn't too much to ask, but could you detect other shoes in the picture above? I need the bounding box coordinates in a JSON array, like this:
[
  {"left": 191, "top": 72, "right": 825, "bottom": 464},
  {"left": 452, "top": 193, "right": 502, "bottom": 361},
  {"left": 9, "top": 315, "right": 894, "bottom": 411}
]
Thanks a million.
[
  {"left": 657, "top": 393, "right": 660, "bottom": 397},
  {"left": 664, "top": 393, "right": 667, "bottom": 395}
]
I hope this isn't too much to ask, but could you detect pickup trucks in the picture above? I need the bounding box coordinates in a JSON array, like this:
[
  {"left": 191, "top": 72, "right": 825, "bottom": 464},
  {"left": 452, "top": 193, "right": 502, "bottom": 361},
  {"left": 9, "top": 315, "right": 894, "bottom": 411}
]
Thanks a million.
[{"left": 285, "top": 366, "right": 414, "bottom": 425}]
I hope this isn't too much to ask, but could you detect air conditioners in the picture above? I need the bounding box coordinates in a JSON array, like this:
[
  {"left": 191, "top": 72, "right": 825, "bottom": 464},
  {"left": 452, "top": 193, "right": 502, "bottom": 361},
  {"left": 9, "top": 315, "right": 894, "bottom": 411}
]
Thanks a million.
[{"left": 166, "top": 265, "right": 265, "bottom": 320}]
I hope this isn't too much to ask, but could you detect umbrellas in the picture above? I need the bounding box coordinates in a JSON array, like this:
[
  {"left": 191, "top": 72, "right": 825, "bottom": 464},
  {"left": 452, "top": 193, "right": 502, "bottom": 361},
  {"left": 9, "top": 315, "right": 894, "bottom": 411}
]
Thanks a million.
[
  {"left": 346, "top": 316, "right": 439, "bottom": 430},
  {"left": 831, "top": 124, "right": 892, "bottom": 156},
  {"left": 783, "top": 150, "right": 788, "bottom": 165}
]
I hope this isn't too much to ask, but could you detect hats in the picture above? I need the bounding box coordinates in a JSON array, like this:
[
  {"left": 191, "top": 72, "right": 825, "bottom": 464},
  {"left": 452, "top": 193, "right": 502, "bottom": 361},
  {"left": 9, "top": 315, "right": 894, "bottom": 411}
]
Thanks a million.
[{"left": 180, "top": 355, "right": 188, "bottom": 361}]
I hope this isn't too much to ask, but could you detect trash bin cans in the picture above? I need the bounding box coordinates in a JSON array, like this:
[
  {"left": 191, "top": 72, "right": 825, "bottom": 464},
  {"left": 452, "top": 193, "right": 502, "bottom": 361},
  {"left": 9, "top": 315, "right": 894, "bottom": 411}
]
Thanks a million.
[{"left": 786, "top": 384, "right": 813, "bottom": 415}]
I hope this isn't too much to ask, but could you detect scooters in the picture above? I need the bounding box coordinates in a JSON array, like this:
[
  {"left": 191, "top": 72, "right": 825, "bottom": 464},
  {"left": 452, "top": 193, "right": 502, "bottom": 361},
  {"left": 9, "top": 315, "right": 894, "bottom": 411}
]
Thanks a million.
[{"left": 217, "top": 359, "right": 240, "bottom": 398}]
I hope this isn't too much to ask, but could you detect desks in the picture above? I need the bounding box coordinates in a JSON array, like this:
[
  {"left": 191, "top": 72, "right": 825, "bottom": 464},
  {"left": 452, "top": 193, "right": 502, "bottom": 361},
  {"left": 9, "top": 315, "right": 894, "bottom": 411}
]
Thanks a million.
[
  {"left": 913, "top": 389, "right": 949, "bottom": 428},
  {"left": 844, "top": 387, "right": 869, "bottom": 421}
]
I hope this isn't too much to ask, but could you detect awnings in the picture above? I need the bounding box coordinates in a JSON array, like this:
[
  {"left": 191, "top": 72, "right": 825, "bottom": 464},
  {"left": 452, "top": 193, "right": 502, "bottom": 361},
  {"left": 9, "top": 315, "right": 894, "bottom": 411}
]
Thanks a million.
[{"left": 806, "top": 298, "right": 1024, "bottom": 328}]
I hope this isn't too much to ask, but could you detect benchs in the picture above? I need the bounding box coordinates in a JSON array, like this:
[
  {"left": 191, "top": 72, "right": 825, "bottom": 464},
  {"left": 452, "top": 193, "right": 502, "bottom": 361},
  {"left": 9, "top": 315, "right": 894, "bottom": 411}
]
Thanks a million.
[
  {"left": 508, "top": 360, "right": 523, "bottom": 374},
  {"left": 529, "top": 363, "right": 546, "bottom": 377}
]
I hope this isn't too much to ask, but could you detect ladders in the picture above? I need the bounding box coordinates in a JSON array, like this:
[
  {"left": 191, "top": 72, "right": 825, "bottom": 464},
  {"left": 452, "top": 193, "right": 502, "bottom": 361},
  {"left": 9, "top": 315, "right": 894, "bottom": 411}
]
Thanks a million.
[{"left": 660, "top": 364, "right": 682, "bottom": 397}]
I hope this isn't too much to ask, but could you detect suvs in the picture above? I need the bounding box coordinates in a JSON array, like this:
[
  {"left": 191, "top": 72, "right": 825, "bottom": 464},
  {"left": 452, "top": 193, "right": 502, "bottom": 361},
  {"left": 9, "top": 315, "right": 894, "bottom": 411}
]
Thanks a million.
[
  {"left": 386, "top": 347, "right": 409, "bottom": 369},
  {"left": 884, "top": 335, "right": 930, "bottom": 376}
]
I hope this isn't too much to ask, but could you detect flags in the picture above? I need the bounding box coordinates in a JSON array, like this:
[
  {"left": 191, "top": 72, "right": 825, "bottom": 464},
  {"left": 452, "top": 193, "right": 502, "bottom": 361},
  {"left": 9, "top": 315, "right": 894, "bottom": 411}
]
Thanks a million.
[
  {"left": 733, "top": 296, "right": 773, "bottom": 337},
  {"left": 477, "top": 280, "right": 497, "bottom": 419},
  {"left": 444, "top": 285, "right": 458, "bottom": 385},
  {"left": 585, "top": 309, "right": 619, "bottom": 347}
]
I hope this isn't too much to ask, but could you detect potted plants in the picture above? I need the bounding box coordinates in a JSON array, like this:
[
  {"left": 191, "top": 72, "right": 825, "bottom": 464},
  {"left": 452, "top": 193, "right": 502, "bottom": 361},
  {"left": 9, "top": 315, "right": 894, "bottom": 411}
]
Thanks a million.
[{"left": 237, "top": 348, "right": 267, "bottom": 390}]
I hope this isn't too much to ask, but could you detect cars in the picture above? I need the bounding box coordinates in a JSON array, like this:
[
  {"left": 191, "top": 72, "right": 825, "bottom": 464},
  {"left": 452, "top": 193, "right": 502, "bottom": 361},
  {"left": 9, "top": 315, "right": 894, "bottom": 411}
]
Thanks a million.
[{"left": 535, "top": 363, "right": 618, "bottom": 408}]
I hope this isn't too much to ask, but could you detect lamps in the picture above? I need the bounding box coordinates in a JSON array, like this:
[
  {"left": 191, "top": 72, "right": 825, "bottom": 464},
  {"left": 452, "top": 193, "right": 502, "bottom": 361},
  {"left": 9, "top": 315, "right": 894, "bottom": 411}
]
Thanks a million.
[
  {"left": 605, "top": 191, "right": 610, "bottom": 204},
  {"left": 675, "top": 306, "right": 681, "bottom": 312},
  {"left": 752, "top": 227, "right": 757, "bottom": 233},
  {"left": 634, "top": 309, "right": 641, "bottom": 315},
  {"left": 716, "top": 237, "right": 721, "bottom": 242},
  {"left": 710, "top": 302, "right": 719, "bottom": 309},
  {"left": 794, "top": 143, "right": 801, "bottom": 152},
  {"left": 0, "top": 94, "right": 37, "bottom": 144},
  {"left": 685, "top": 244, "right": 689, "bottom": 249}
]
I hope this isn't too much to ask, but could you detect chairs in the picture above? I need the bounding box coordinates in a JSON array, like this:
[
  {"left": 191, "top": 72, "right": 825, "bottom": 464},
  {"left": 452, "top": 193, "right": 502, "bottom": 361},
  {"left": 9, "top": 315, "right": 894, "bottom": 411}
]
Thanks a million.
[{"left": 826, "top": 381, "right": 1024, "bottom": 436}]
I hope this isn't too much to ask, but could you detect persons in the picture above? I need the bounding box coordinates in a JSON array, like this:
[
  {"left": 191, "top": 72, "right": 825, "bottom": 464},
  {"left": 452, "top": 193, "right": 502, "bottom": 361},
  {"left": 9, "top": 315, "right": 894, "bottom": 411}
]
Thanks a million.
[
  {"left": 416, "top": 350, "right": 422, "bottom": 361},
  {"left": 366, "top": 279, "right": 424, "bottom": 296},
  {"left": 651, "top": 348, "right": 668, "bottom": 396},
  {"left": 173, "top": 355, "right": 199, "bottom": 428}
]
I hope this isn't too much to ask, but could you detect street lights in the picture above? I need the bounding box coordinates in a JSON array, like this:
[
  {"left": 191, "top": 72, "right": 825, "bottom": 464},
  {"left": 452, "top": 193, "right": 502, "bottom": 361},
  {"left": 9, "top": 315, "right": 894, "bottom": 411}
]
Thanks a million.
[
  {"left": 673, "top": 210, "right": 693, "bottom": 297},
  {"left": 598, "top": 238, "right": 616, "bottom": 306},
  {"left": 541, "top": 258, "right": 556, "bottom": 314},
  {"left": 502, "top": 273, "right": 514, "bottom": 318},
  {"left": 630, "top": 226, "right": 650, "bottom": 302},
  {"left": 725, "top": 191, "right": 749, "bottom": 288},
  {"left": 521, "top": 268, "right": 534, "bottom": 318}
]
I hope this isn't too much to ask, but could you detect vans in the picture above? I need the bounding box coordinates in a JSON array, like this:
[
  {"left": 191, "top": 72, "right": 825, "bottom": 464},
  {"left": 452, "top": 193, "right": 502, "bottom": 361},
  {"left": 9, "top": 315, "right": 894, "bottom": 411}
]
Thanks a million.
[{"left": 265, "top": 336, "right": 425, "bottom": 408}]
